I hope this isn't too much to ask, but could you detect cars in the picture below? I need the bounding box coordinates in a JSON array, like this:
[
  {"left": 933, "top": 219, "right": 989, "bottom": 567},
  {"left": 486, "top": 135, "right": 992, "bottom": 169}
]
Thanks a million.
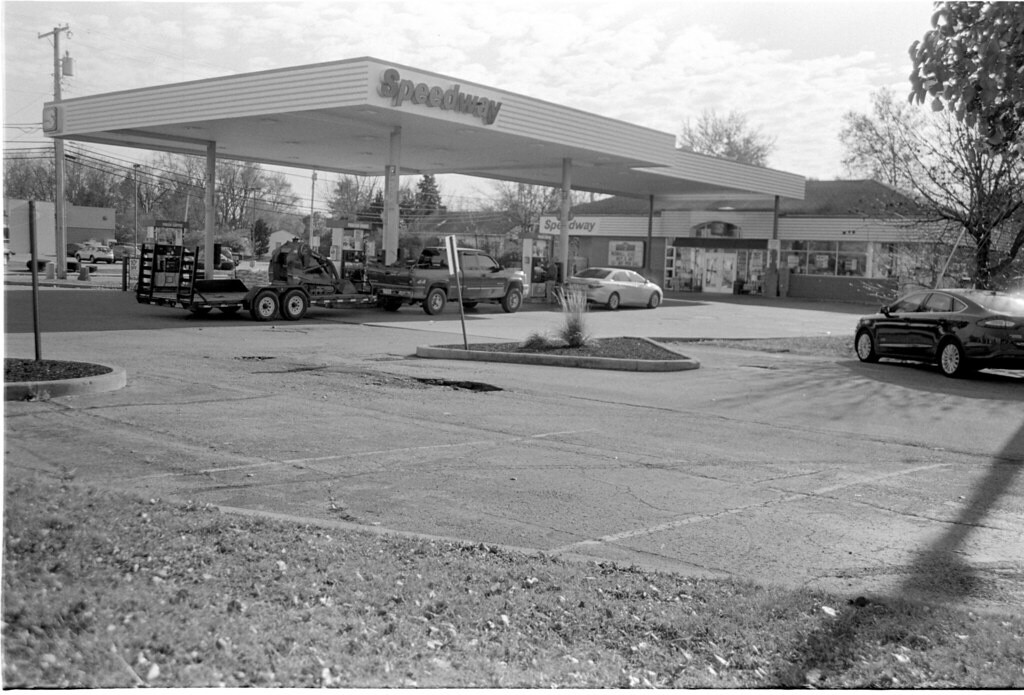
[
  {"left": 561, "top": 266, "right": 664, "bottom": 311},
  {"left": 493, "top": 266, "right": 547, "bottom": 300},
  {"left": 113, "top": 245, "right": 141, "bottom": 262},
  {"left": 184, "top": 241, "right": 240, "bottom": 271},
  {"left": 854, "top": 287, "right": 1024, "bottom": 381},
  {"left": 66, "top": 238, "right": 142, "bottom": 255},
  {"left": 75, "top": 245, "right": 115, "bottom": 264}
]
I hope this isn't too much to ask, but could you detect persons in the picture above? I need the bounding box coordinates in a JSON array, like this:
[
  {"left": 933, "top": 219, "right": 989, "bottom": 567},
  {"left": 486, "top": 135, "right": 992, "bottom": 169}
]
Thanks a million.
[{"left": 539, "top": 258, "right": 559, "bottom": 304}]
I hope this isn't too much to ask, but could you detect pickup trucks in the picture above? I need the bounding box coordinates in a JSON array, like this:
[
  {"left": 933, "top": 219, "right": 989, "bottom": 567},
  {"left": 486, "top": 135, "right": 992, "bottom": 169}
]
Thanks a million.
[{"left": 363, "top": 247, "right": 530, "bottom": 317}]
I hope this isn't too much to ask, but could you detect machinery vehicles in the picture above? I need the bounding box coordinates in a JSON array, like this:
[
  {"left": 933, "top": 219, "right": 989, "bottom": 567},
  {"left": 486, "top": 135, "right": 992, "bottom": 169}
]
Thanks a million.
[{"left": 136, "top": 219, "right": 376, "bottom": 322}]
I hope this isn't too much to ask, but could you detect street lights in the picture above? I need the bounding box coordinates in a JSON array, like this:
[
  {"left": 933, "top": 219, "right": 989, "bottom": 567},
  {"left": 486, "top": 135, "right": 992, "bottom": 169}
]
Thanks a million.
[
  {"left": 247, "top": 185, "right": 262, "bottom": 268},
  {"left": 131, "top": 161, "right": 143, "bottom": 259}
]
